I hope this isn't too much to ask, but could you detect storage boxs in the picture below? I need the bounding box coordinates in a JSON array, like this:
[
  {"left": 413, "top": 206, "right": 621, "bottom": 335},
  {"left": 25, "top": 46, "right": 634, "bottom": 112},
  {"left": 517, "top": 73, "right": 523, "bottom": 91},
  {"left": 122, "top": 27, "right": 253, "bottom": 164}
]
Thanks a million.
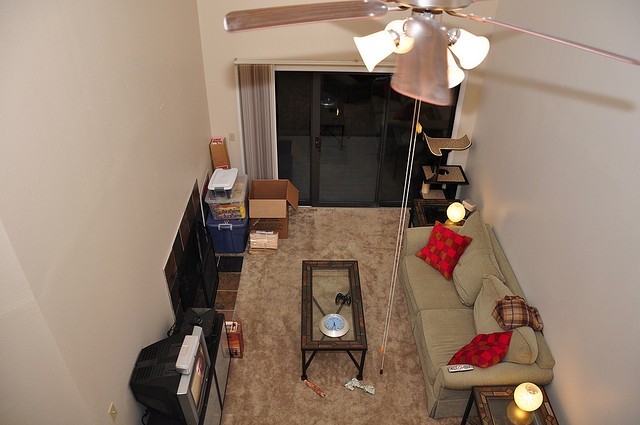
[
  {"left": 207, "top": 213, "right": 250, "bottom": 253},
  {"left": 249, "top": 179, "right": 299, "bottom": 242},
  {"left": 207, "top": 167, "right": 239, "bottom": 199},
  {"left": 278, "top": 139, "right": 303, "bottom": 183},
  {"left": 204, "top": 174, "right": 248, "bottom": 220}
]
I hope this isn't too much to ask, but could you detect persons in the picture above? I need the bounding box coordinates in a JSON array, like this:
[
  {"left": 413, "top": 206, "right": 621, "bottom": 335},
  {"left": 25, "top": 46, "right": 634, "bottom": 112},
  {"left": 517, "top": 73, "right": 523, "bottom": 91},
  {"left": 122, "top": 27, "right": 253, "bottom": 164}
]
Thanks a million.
[{"left": 196, "top": 355, "right": 205, "bottom": 383}]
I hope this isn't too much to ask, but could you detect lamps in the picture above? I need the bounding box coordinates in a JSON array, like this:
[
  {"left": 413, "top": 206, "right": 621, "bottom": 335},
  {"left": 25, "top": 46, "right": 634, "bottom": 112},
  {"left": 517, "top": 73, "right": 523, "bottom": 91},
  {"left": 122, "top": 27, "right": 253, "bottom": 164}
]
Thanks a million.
[
  {"left": 447, "top": 202, "right": 467, "bottom": 222},
  {"left": 351, "top": 2, "right": 490, "bottom": 90},
  {"left": 514, "top": 381, "right": 544, "bottom": 413},
  {"left": 506, "top": 399, "right": 535, "bottom": 425}
]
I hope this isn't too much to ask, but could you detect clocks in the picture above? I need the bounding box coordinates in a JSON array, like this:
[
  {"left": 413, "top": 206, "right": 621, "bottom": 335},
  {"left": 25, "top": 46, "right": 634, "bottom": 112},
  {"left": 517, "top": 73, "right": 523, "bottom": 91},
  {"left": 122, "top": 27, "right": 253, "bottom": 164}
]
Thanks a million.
[
  {"left": 400, "top": 210, "right": 555, "bottom": 419},
  {"left": 318, "top": 313, "right": 349, "bottom": 337}
]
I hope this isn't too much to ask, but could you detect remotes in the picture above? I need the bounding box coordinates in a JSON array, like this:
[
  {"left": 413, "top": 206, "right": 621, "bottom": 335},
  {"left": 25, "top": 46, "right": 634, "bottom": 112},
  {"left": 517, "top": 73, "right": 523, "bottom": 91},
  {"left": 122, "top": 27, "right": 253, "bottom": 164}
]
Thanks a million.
[{"left": 447, "top": 364, "right": 474, "bottom": 373}]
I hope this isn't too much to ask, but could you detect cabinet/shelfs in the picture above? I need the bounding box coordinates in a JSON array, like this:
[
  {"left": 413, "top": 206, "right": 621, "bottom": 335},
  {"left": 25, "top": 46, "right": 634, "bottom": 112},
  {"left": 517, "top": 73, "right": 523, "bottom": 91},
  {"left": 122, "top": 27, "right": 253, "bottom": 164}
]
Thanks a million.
[
  {"left": 147, "top": 313, "right": 232, "bottom": 424},
  {"left": 423, "top": 132, "right": 472, "bottom": 201}
]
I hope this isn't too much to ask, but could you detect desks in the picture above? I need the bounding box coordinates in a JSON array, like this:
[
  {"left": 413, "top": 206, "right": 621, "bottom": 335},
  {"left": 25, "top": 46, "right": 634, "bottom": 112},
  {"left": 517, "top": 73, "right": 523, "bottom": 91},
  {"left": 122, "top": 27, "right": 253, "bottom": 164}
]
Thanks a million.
[
  {"left": 319, "top": 83, "right": 345, "bottom": 149},
  {"left": 408, "top": 197, "right": 462, "bottom": 227},
  {"left": 460, "top": 385, "right": 558, "bottom": 425}
]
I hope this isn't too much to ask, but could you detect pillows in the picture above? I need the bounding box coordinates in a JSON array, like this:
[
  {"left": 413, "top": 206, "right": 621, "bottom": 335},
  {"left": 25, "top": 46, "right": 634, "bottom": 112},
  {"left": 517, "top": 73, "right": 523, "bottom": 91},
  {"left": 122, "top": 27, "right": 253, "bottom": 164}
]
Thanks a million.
[
  {"left": 448, "top": 332, "right": 512, "bottom": 368},
  {"left": 473, "top": 274, "right": 540, "bottom": 366},
  {"left": 392, "top": 101, "right": 413, "bottom": 121},
  {"left": 378, "top": 81, "right": 402, "bottom": 102},
  {"left": 452, "top": 211, "right": 507, "bottom": 307},
  {"left": 414, "top": 219, "right": 473, "bottom": 281}
]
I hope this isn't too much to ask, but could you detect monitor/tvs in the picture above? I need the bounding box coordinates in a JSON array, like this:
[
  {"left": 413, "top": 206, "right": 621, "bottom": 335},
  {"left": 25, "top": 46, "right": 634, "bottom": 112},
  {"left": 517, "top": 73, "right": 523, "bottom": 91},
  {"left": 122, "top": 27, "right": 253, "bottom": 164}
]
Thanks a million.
[
  {"left": 162, "top": 178, "right": 219, "bottom": 331},
  {"left": 128, "top": 327, "right": 211, "bottom": 425}
]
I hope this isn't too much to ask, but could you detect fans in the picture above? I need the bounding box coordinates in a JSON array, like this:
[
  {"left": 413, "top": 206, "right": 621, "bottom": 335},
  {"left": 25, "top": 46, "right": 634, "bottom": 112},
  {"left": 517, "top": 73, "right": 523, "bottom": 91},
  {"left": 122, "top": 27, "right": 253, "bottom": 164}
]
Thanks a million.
[{"left": 224, "top": 0, "right": 639, "bottom": 107}]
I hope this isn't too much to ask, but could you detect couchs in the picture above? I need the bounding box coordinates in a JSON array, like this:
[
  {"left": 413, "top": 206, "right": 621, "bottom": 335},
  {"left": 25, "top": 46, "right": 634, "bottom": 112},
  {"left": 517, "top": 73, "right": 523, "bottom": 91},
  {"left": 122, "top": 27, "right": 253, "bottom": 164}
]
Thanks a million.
[{"left": 370, "top": 79, "right": 450, "bottom": 133}]
